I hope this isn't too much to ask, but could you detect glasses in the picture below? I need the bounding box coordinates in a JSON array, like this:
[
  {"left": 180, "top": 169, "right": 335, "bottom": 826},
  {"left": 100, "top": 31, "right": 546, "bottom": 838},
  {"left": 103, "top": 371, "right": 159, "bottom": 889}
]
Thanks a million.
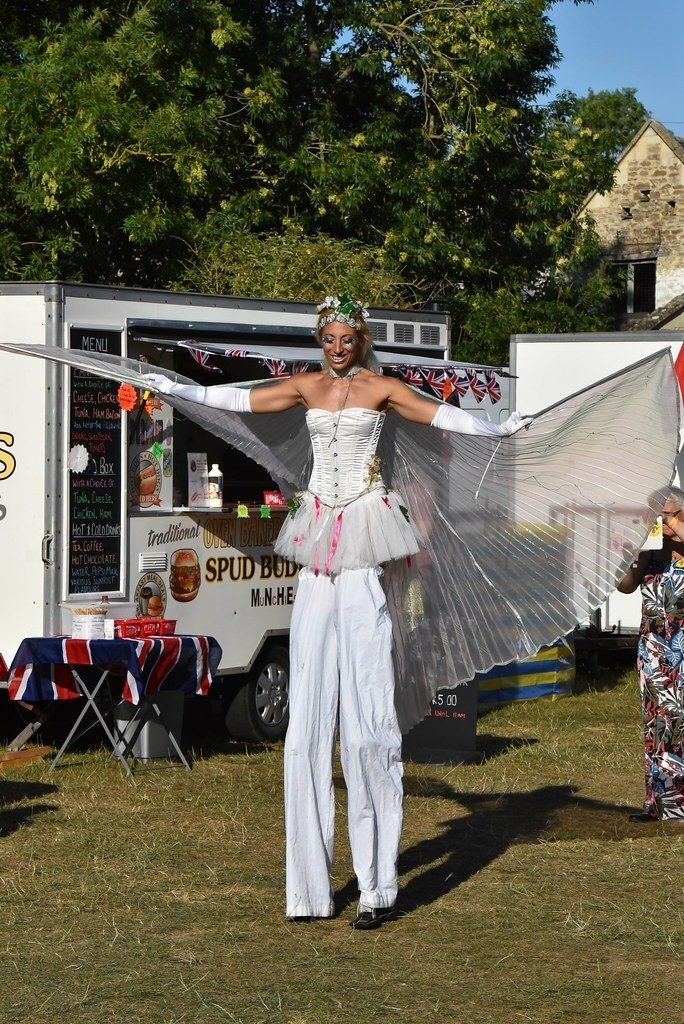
[{"left": 665, "top": 510, "right": 681, "bottom": 516}]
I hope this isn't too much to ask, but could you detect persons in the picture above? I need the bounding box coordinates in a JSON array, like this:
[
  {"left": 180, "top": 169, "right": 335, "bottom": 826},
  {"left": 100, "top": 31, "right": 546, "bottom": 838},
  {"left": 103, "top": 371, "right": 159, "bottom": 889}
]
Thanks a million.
[
  {"left": 614, "top": 485, "right": 684, "bottom": 822},
  {"left": 147, "top": 295, "right": 537, "bottom": 751}
]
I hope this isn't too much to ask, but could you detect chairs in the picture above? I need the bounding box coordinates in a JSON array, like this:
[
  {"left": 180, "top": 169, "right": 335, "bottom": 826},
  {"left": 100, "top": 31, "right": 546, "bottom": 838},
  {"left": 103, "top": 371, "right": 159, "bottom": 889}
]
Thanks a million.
[{"left": 0, "top": 652, "right": 56, "bottom": 750}]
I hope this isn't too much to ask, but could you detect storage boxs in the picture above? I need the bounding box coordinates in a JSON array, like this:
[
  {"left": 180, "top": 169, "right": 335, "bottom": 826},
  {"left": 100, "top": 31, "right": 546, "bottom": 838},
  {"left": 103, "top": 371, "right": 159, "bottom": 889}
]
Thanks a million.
[
  {"left": 114, "top": 618, "right": 178, "bottom": 638},
  {"left": 111, "top": 689, "right": 184, "bottom": 758}
]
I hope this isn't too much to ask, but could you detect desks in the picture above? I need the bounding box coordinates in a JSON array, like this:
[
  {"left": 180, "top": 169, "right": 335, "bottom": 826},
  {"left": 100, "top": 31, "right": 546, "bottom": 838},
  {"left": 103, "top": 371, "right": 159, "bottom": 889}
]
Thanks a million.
[{"left": 7, "top": 634, "right": 223, "bottom": 778}]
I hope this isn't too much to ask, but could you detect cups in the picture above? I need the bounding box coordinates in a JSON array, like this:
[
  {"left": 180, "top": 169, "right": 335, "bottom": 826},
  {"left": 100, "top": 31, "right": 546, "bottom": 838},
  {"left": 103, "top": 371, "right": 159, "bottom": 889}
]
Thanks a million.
[{"left": 141, "top": 587, "right": 152, "bottom": 615}]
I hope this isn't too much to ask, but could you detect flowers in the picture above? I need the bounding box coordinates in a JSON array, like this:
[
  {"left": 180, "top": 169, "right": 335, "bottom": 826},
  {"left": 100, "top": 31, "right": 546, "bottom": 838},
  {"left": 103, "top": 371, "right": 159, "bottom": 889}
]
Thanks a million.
[{"left": 313, "top": 292, "right": 370, "bottom": 329}]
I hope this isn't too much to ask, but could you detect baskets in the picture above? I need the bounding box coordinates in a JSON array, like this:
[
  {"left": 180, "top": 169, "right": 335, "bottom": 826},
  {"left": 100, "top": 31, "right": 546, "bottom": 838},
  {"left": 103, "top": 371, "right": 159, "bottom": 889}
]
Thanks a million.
[{"left": 112, "top": 616, "right": 177, "bottom": 638}]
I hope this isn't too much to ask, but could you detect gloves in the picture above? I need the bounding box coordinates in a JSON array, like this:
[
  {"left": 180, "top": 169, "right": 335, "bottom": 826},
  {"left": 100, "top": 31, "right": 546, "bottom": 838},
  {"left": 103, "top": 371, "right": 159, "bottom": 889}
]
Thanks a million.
[
  {"left": 430, "top": 403, "right": 533, "bottom": 437},
  {"left": 137, "top": 373, "right": 253, "bottom": 414}
]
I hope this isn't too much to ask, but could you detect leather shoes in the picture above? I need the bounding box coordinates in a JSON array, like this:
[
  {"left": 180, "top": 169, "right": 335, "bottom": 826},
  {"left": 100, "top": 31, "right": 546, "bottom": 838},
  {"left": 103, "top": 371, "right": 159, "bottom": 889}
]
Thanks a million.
[{"left": 629, "top": 814, "right": 655, "bottom": 823}]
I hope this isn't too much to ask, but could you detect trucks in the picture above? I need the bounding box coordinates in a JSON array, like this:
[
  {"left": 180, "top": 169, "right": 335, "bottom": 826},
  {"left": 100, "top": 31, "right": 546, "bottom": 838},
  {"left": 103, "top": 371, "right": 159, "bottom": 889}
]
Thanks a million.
[
  {"left": 0, "top": 280, "right": 511, "bottom": 770},
  {"left": 510, "top": 331, "right": 684, "bottom": 692}
]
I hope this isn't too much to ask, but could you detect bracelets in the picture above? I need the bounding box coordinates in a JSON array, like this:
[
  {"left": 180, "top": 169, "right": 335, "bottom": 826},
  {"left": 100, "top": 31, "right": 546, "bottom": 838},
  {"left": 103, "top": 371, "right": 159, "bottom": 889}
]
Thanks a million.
[{"left": 667, "top": 516, "right": 678, "bottom": 529}]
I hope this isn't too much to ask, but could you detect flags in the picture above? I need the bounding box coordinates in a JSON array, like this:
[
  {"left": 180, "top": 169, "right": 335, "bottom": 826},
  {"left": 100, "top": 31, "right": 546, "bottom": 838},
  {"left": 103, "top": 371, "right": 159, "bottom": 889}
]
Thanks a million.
[
  {"left": 465, "top": 367, "right": 485, "bottom": 404},
  {"left": 398, "top": 368, "right": 423, "bottom": 389},
  {"left": 419, "top": 368, "right": 446, "bottom": 400},
  {"left": 445, "top": 367, "right": 470, "bottom": 398},
  {"left": 485, "top": 369, "right": 504, "bottom": 404},
  {"left": 494, "top": 370, "right": 517, "bottom": 380},
  {"left": 184, "top": 338, "right": 310, "bottom": 378}
]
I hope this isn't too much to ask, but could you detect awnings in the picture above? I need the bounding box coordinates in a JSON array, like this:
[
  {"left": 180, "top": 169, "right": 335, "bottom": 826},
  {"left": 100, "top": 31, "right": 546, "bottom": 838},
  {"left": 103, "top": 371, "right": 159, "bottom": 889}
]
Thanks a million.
[{"left": 136, "top": 329, "right": 501, "bottom": 371}]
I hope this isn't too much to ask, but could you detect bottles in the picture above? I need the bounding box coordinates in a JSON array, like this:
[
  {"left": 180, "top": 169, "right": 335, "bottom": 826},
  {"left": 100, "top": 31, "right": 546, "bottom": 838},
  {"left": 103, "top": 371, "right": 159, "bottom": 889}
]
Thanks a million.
[
  {"left": 100, "top": 596, "right": 112, "bottom": 619},
  {"left": 137, "top": 418, "right": 170, "bottom": 477},
  {"left": 208, "top": 463, "right": 223, "bottom": 508},
  {"left": 134, "top": 572, "right": 166, "bottom": 619},
  {"left": 103, "top": 619, "right": 114, "bottom": 640}
]
